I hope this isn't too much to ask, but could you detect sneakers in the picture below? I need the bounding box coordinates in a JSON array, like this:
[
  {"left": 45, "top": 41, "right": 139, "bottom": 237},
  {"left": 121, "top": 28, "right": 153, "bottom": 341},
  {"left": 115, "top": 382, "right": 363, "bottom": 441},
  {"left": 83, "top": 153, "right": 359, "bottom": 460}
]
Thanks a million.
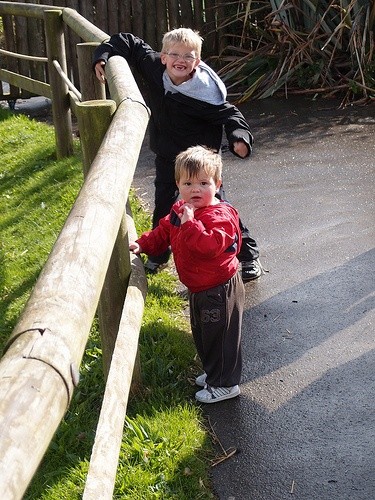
[
  {"left": 144, "top": 260, "right": 169, "bottom": 275},
  {"left": 195, "top": 383, "right": 241, "bottom": 403},
  {"left": 195, "top": 373, "right": 207, "bottom": 386},
  {"left": 241, "top": 257, "right": 269, "bottom": 281}
]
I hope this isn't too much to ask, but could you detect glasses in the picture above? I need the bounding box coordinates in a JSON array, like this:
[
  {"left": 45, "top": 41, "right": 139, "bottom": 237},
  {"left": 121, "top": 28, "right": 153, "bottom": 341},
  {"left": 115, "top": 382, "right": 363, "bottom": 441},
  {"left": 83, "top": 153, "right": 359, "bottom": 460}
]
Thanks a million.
[{"left": 163, "top": 52, "right": 198, "bottom": 60}]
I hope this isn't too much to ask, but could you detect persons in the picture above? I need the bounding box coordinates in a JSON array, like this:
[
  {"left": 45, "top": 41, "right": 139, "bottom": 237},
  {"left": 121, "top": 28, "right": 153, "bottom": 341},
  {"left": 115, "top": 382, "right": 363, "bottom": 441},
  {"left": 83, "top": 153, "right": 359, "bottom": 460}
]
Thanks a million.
[
  {"left": 128, "top": 145, "right": 246, "bottom": 403},
  {"left": 92, "top": 27, "right": 262, "bottom": 281}
]
